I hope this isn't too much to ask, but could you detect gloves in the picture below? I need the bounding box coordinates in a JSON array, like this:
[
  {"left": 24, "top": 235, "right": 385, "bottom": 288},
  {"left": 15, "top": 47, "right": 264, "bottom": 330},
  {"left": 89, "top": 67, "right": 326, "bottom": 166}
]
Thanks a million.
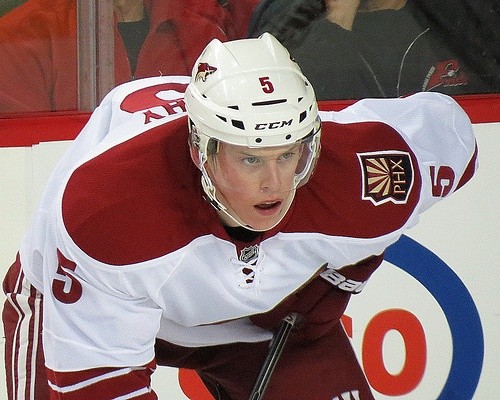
[{"left": 249, "top": 253, "right": 385, "bottom": 342}]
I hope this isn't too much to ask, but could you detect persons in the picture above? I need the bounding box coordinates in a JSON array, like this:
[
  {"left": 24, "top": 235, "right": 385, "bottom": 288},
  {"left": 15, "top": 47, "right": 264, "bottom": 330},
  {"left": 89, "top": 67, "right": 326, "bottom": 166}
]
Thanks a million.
[
  {"left": 249, "top": 0, "right": 499, "bottom": 101},
  {"left": 1, "top": 0, "right": 268, "bottom": 122},
  {"left": 0, "top": 32, "right": 482, "bottom": 400}
]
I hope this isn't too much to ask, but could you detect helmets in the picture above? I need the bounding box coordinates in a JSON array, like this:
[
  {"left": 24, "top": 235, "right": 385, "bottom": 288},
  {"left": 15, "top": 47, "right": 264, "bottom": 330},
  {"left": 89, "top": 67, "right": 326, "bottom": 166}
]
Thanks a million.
[{"left": 183, "top": 32, "right": 322, "bottom": 155}]
